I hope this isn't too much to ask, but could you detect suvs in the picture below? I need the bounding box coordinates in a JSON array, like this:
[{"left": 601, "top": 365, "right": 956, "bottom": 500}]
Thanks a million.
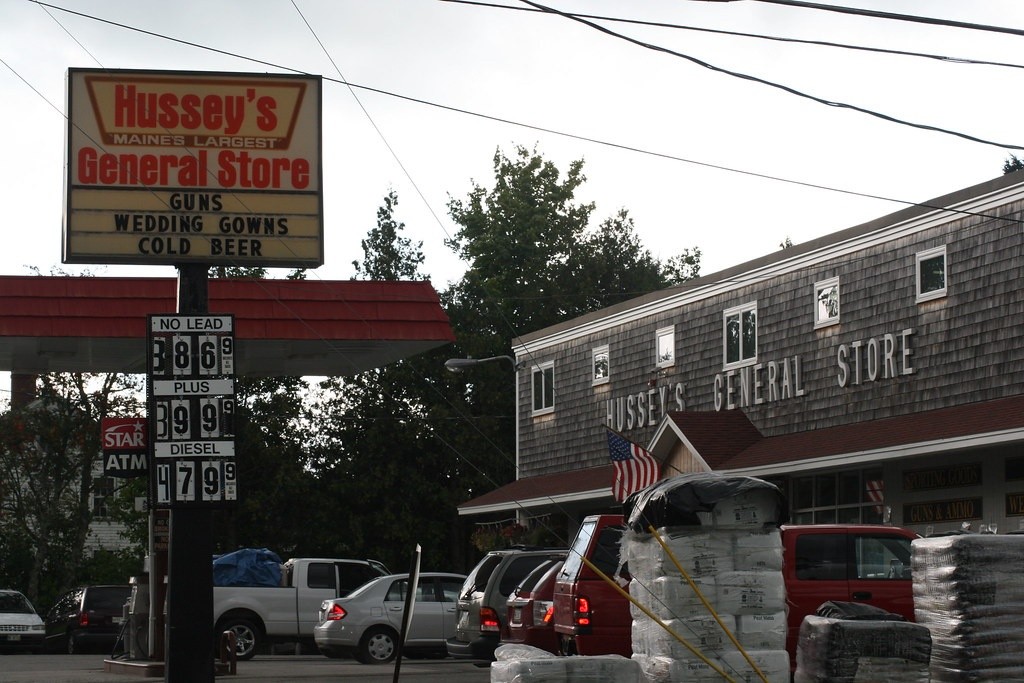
[{"left": 444, "top": 544, "right": 568, "bottom": 668}]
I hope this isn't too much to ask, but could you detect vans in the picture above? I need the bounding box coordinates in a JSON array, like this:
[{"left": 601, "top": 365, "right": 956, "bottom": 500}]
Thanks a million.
[
  {"left": 552, "top": 514, "right": 924, "bottom": 671},
  {"left": 44, "top": 584, "right": 137, "bottom": 654}
]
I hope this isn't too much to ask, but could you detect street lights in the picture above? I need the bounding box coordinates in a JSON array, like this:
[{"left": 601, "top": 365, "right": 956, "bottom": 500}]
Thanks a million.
[{"left": 444, "top": 355, "right": 517, "bottom": 373}]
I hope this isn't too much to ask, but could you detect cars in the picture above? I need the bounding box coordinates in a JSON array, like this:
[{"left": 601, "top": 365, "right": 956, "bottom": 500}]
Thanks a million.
[
  {"left": 313, "top": 572, "right": 469, "bottom": 665},
  {"left": 0, "top": 590, "right": 46, "bottom": 651},
  {"left": 498, "top": 557, "right": 567, "bottom": 654}
]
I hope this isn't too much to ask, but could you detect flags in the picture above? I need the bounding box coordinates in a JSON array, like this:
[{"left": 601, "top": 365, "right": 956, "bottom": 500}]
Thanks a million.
[
  {"left": 604, "top": 428, "right": 658, "bottom": 502},
  {"left": 867, "top": 478, "right": 887, "bottom": 515}
]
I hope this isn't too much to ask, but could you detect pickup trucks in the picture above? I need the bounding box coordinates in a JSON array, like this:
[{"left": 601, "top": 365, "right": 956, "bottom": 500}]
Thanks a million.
[{"left": 213, "top": 557, "right": 394, "bottom": 661}]
